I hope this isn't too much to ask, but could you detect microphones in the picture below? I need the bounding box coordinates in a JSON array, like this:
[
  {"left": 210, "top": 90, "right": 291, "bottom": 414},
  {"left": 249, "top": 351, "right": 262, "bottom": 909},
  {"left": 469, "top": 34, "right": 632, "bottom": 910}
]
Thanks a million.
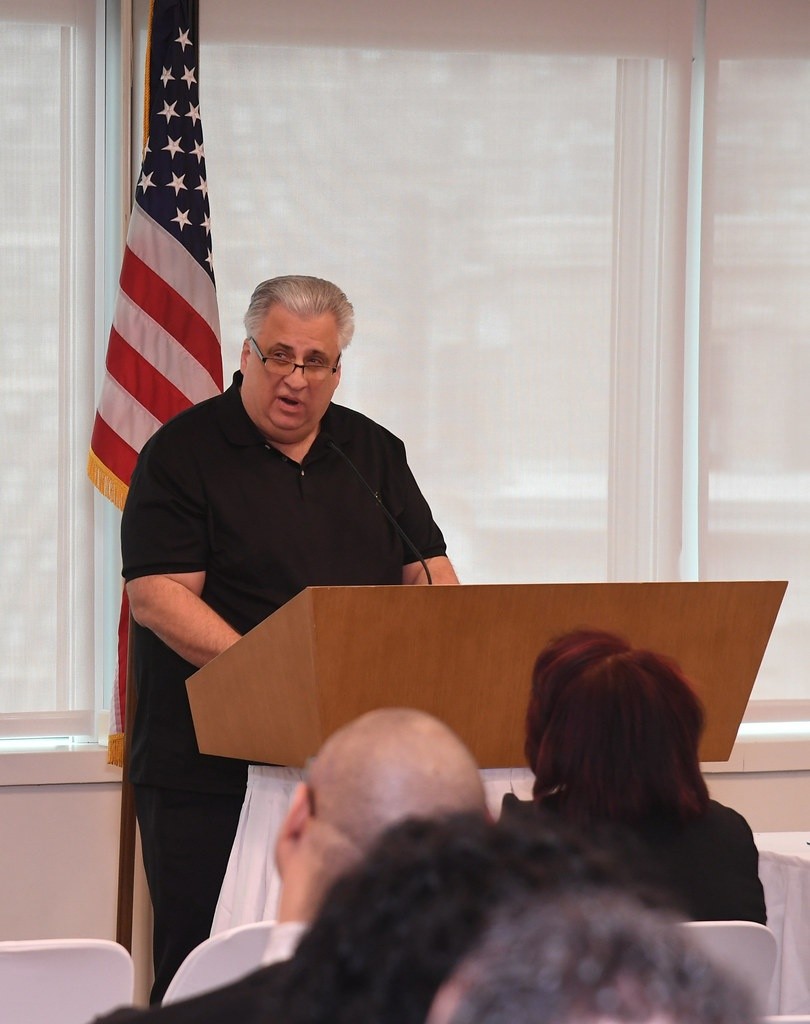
[{"left": 318, "top": 432, "right": 432, "bottom": 586}]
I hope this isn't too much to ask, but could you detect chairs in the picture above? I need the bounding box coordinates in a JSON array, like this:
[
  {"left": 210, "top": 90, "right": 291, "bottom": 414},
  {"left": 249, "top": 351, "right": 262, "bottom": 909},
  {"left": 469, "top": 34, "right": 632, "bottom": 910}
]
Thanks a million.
[
  {"left": 0, "top": 935, "right": 137, "bottom": 1024},
  {"left": 160, "top": 920, "right": 275, "bottom": 1004},
  {"left": 676, "top": 919, "right": 777, "bottom": 1016}
]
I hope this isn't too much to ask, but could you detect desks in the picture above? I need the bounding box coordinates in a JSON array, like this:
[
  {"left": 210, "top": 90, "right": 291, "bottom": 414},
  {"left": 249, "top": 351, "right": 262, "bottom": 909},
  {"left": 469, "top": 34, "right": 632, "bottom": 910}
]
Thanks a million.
[{"left": 752, "top": 830, "right": 810, "bottom": 1015}]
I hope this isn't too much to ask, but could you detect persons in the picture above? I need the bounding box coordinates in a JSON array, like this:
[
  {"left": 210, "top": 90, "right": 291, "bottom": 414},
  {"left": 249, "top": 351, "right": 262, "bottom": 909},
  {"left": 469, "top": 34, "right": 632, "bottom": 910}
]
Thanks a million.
[
  {"left": 497, "top": 627, "right": 767, "bottom": 924},
  {"left": 121, "top": 276, "right": 459, "bottom": 1006},
  {"left": 89, "top": 710, "right": 760, "bottom": 1024}
]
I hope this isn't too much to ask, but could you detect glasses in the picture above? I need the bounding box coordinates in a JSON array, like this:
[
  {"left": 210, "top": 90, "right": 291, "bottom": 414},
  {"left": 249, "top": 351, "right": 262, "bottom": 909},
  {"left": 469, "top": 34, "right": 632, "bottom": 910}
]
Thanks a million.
[{"left": 246, "top": 336, "right": 343, "bottom": 382}]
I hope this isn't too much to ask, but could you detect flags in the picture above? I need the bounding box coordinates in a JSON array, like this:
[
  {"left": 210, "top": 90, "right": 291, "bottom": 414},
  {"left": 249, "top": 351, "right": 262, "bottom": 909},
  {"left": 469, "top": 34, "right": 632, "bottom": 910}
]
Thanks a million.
[{"left": 87, "top": 1, "right": 225, "bottom": 766}]
